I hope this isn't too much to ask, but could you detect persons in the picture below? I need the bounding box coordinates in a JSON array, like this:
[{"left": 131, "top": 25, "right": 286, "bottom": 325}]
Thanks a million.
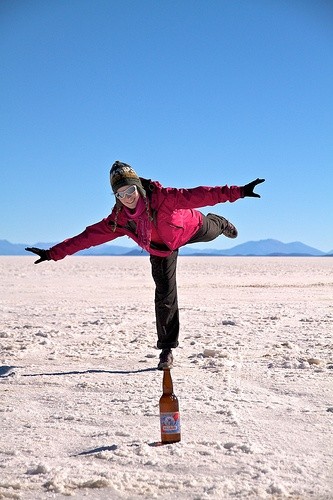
[{"left": 25, "top": 160, "right": 266, "bottom": 371}]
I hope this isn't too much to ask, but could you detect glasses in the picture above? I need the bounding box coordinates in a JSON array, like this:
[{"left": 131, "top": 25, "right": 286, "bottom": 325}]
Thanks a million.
[{"left": 116, "top": 185, "right": 137, "bottom": 199}]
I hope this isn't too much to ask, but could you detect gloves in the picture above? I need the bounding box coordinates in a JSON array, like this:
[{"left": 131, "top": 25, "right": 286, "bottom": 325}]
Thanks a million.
[
  {"left": 25, "top": 246, "right": 51, "bottom": 267},
  {"left": 243, "top": 178, "right": 266, "bottom": 198}
]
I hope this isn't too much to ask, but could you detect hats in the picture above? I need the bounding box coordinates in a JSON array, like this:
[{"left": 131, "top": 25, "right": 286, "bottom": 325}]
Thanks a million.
[{"left": 109, "top": 161, "right": 154, "bottom": 232}]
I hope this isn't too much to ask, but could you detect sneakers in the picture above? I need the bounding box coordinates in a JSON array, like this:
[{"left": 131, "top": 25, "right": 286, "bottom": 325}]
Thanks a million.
[
  {"left": 223, "top": 222, "right": 238, "bottom": 238},
  {"left": 158, "top": 350, "right": 173, "bottom": 370}
]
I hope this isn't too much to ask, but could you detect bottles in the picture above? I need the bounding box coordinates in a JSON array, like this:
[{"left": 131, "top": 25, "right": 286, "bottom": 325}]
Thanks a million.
[{"left": 159, "top": 368, "right": 181, "bottom": 443}]
